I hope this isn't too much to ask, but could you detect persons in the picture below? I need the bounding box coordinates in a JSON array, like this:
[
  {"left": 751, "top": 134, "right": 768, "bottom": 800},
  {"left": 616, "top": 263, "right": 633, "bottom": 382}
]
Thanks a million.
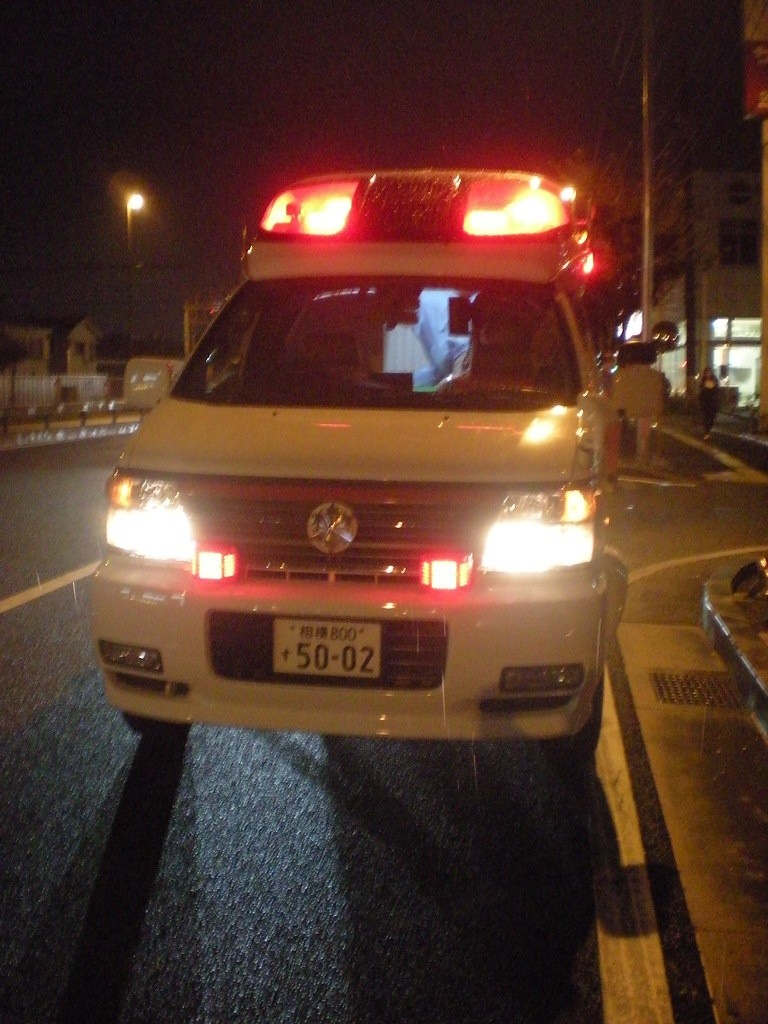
[
  {"left": 661, "top": 372, "right": 672, "bottom": 404},
  {"left": 698, "top": 367, "right": 723, "bottom": 440},
  {"left": 436, "top": 315, "right": 577, "bottom": 406}
]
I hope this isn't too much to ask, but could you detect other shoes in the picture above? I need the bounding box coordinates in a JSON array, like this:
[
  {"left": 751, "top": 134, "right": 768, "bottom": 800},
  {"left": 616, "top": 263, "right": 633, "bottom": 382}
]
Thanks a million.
[{"left": 704, "top": 434, "right": 709, "bottom": 439}]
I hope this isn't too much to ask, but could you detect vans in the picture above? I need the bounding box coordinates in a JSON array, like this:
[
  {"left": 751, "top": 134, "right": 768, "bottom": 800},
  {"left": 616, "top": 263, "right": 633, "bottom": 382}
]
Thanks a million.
[{"left": 91, "top": 172, "right": 607, "bottom": 770}]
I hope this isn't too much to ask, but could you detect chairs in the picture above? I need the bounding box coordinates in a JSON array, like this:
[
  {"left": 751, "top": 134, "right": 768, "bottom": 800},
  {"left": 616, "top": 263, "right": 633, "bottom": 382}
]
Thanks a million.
[{"left": 286, "top": 305, "right": 365, "bottom": 382}]
